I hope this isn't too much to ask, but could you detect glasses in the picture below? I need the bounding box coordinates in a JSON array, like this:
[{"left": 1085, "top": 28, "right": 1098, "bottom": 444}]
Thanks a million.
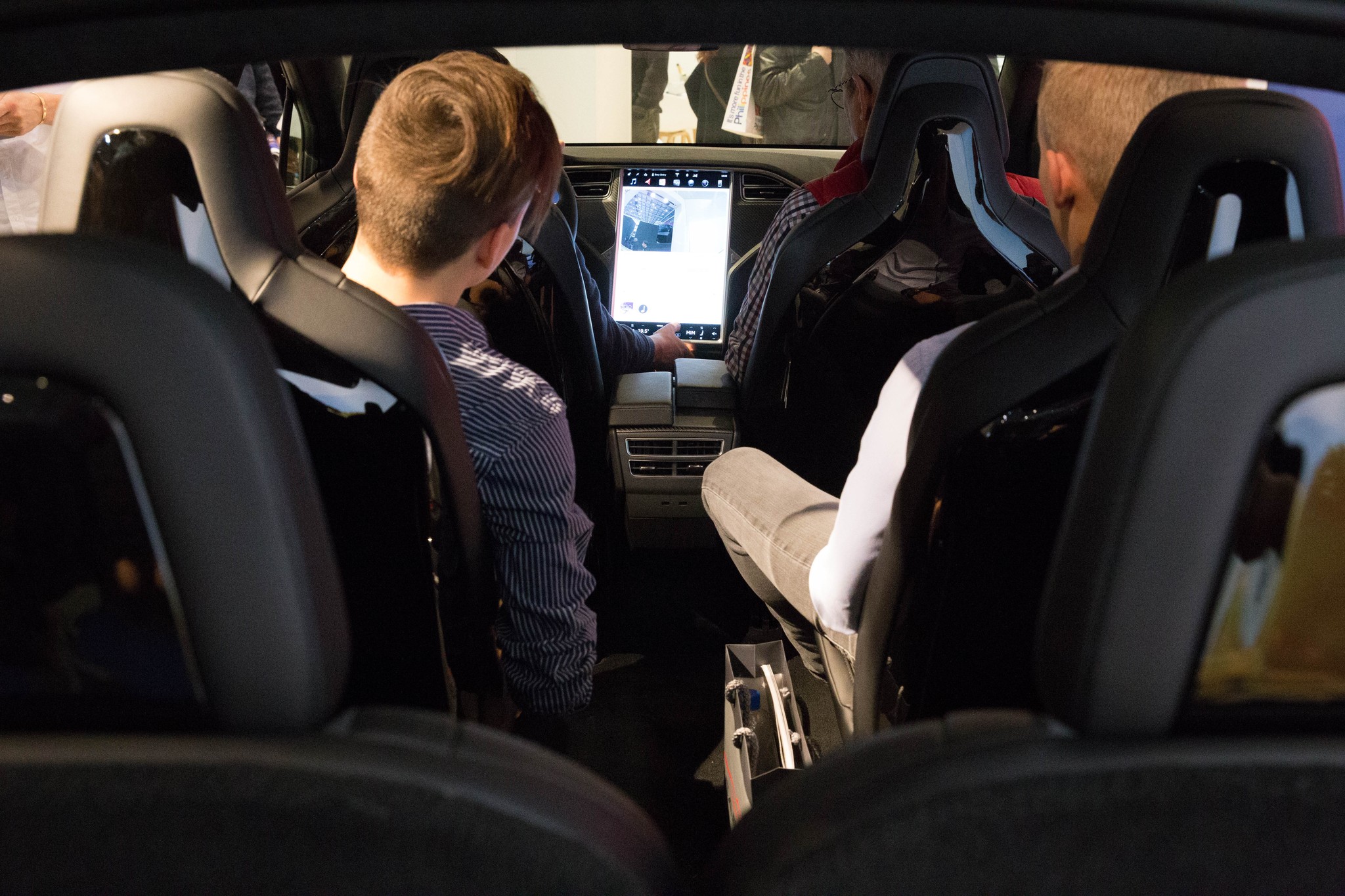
[{"left": 827, "top": 77, "right": 853, "bottom": 110}]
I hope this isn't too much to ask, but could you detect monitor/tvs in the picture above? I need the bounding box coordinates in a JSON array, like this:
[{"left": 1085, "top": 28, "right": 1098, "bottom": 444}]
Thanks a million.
[{"left": 608, "top": 166, "right": 733, "bottom": 343}]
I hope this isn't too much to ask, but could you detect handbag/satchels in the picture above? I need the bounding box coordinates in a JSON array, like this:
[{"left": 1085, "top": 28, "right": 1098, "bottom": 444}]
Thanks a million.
[
  {"left": 723, "top": 639, "right": 812, "bottom": 830},
  {"left": 721, "top": 43, "right": 764, "bottom": 138}
]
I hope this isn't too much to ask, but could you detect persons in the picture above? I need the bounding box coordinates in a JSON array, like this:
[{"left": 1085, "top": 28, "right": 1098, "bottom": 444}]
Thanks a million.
[
  {"left": 341, "top": 49, "right": 598, "bottom": 718},
  {"left": 701, "top": 59, "right": 1248, "bottom": 680},
  {"left": 0, "top": 45, "right": 856, "bottom": 371},
  {"left": 723, "top": 48, "right": 1050, "bottom": 387}
]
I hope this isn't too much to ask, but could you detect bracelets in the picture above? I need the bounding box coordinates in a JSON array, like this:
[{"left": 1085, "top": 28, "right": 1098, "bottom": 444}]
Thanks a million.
[{"left": 29, "top": 92, "right": 46, "bottom": 125}]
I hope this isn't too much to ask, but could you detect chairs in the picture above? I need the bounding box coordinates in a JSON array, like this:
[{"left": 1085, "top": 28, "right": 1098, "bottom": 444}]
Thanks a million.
[{"left": 0, "top": 44, "right": 1345, "bottom": 896}]
